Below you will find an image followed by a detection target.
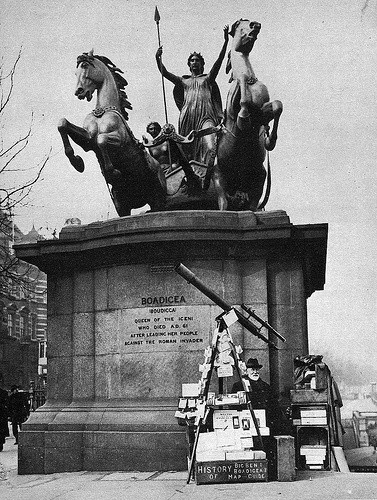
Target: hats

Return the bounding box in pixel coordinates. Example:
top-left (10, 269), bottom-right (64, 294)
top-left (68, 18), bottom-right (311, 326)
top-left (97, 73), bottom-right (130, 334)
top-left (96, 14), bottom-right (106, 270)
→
top-left (246, 358), bottom-right (263, 369)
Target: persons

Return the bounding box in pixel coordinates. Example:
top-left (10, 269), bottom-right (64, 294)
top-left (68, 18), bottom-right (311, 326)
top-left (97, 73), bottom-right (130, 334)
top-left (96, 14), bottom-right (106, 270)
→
top-left (230, 358), bottom-right (278, 432)
top-left (0, 388), bottom-right (9, 452)
top-left (142, 122), bottom-right (190, 171)
top-left (155, 23), bottom-right (230, 209)
top-left (8, 385), bottom-right (31, 445)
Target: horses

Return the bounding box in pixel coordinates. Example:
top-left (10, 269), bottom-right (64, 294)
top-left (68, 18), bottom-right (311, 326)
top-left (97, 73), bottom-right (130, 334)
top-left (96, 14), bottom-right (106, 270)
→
top-left (57, 47), bottom-right (168, 218)
top-left (215, 17), bottom-right (283, 212)
